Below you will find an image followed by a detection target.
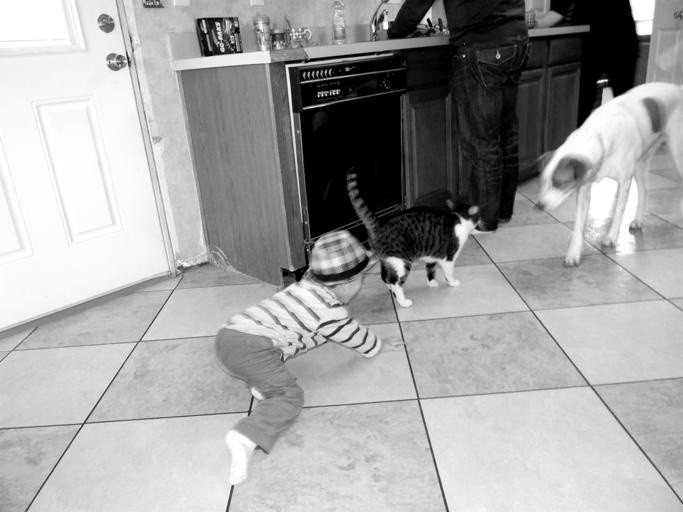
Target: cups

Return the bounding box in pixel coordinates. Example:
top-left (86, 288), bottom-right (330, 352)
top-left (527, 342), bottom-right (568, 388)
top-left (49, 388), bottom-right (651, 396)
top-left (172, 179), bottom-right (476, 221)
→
top-left (524, 11), bottom-right (535, 28)
top-left (285, 27), bottom-right (312, 49)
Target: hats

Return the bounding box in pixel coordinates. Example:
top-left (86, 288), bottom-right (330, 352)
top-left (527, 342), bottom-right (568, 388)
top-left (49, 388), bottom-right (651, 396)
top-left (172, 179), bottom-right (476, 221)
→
top-left (301, 230), bottom-right (380, 287)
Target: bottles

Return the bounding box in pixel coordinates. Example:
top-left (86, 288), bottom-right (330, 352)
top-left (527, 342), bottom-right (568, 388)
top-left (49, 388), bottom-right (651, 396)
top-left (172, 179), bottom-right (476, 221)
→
top-left (331, 1), bottom-right (347, 43)
top-left (251, 12), bottom-right (272, 52)
top-left (269, 27), bottom-right (285, 50)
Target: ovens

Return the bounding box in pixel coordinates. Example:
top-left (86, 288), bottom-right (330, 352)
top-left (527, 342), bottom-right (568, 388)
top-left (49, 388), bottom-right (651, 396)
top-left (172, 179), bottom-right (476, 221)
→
top-left (285, 54), bottom-right (410, 269)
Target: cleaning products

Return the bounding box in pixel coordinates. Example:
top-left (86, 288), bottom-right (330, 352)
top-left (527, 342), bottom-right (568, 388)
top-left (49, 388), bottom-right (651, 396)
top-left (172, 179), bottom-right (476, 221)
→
top-left (333, 0), bottom-right (347, 45)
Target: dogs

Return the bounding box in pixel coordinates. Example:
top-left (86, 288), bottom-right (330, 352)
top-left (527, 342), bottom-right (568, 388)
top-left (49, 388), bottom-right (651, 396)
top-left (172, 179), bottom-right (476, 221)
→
top-left (533, 82), bottom-right (683, 267)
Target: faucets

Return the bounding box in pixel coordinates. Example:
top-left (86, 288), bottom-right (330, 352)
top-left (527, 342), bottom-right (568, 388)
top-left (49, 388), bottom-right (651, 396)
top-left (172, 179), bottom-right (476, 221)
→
top-left (369, 0), bottom-right (390, 41)
top-left (377, 10), bottom-right (388, 31)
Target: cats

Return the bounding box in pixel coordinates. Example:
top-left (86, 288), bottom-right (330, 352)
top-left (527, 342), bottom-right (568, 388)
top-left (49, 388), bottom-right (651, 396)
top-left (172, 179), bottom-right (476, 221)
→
top-left (346, 165), bottom-right (487, 307)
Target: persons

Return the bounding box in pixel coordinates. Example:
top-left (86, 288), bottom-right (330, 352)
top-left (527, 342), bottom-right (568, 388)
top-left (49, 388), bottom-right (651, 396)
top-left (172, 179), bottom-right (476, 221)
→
top-left (215, 231), bottom-right (406, 485)
top-left (386, 0), bottom-right (531, 236)
top-left (535, 2), bottom-right (640, 126)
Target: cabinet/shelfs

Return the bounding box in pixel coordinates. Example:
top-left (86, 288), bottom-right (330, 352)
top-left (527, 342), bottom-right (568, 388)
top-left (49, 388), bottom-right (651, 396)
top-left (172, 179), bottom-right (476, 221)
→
top-left (403, 87), bottom-right (454, 207)
top-left (452, 35), bottom-right (587, 194)
